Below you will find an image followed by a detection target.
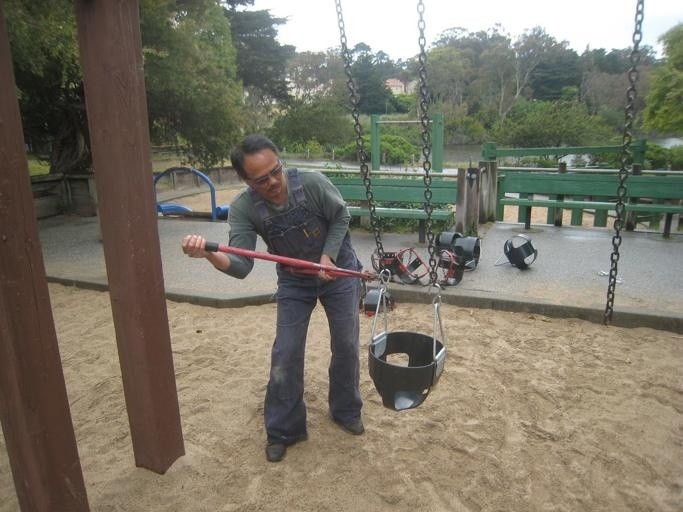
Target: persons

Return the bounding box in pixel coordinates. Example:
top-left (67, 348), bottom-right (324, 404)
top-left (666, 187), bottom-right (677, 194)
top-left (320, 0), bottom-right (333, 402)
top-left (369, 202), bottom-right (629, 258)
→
top-left (180, 133), bottom-right (365, 463)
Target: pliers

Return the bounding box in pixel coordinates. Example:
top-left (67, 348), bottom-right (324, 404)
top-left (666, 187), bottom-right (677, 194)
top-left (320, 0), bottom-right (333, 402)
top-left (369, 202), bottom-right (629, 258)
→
top-left (204, 242), bottom-right (405, 285)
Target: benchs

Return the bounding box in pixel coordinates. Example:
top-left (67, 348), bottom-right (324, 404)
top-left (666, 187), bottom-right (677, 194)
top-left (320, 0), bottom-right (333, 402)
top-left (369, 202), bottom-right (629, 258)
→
top-left (329, 173), bottom-right (460, 243)
top-left (496, 170), bottom-right (681, 238)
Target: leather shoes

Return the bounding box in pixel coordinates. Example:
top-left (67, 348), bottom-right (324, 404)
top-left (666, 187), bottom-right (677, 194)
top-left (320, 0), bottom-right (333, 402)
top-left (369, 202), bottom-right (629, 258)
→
top-left (265, 436), bottom-right (288, 462)
top-left (331, 414), bottom-right (367, 435)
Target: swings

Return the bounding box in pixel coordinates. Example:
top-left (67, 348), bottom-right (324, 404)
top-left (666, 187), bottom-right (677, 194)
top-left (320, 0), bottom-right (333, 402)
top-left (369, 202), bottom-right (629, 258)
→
top-left (333, 0), bottom-right (447, 410)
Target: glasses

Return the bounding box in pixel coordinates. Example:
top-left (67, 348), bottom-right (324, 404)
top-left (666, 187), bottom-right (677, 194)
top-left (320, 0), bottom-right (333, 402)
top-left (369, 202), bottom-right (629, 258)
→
top-left (244, 165), bottom-right (284, 186)
